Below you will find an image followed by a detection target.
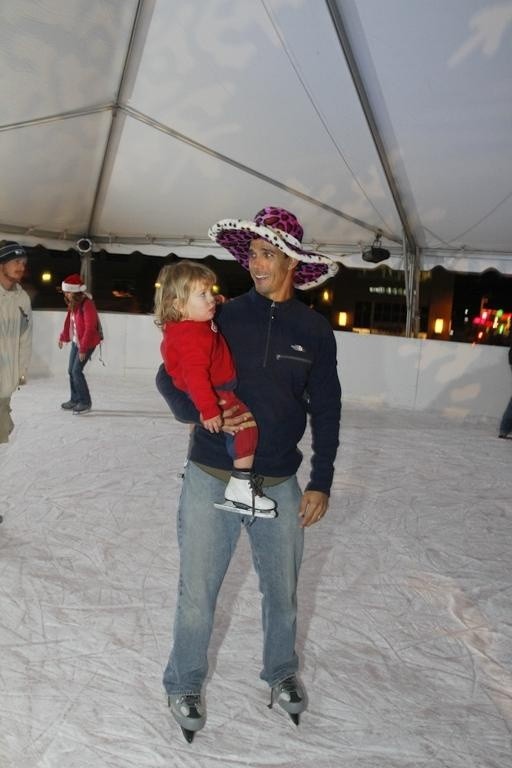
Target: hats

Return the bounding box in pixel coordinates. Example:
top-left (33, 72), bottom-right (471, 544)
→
top-left (0, 238), bottom-right (28, 268)
top-left (206, 204), bottom-right (344, 295)
top-left (61, 272), bottom-right (86, 294)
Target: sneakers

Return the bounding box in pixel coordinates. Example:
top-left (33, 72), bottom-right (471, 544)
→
top-left (168, 694), bottom-right (207, 731)
top-left (271, 677), bottom-right (309, 715)
top-left (62, 395), bottom-right (93, 412)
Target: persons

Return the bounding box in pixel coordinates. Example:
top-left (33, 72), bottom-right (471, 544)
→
top-left (1, 237), bottom-right (34, 527)
top-left (156, 258), bottom-right (281, 513)
top-left (155, 202), bottom-right (344, 732)
top-left (56, 271), bottom-right (104, 414)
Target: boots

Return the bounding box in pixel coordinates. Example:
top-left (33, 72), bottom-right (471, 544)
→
top-left (224, 468), bottom-right (277, 510)
